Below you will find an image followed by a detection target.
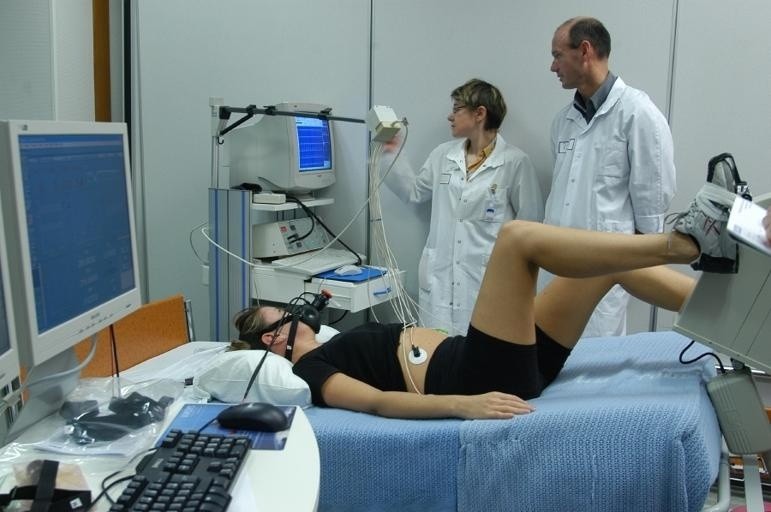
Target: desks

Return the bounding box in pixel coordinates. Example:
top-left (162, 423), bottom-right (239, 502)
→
top-left (0, 403), bottom-right (322, 512)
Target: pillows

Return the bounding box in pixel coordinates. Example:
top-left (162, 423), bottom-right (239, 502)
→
top-left (192, 323), bottom-right (341, 410)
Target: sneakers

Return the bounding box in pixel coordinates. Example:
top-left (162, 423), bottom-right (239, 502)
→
top-left (664, 154), bottom-right (739, 274)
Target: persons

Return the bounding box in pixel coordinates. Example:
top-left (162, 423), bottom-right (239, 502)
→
top-left (231, 152), bottom-right (753, 419)
top-left (534, 15), bottom-right (677, 340)
top-left (761, 203), bottom-right (770, 241)
top-left (376, 79), bottom-right (545, 333)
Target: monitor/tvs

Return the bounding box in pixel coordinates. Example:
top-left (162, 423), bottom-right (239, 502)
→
top-left (222, 101), bottom-right (336, 204)
top-left (1, 117), bottom-right (144, 444)
top-left (1, 216), bottom-right (19, 449)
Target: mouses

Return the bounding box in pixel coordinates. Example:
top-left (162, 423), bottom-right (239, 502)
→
top-left (217, 403), bottom-right (290, 431)
top-left (334, 264), bottom-right (361, 275)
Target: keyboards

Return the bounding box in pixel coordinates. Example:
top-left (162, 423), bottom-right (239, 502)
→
top-left (108, 428), bottom-right (251, 511)
top-left (271, 247), bottom-right (366, 276)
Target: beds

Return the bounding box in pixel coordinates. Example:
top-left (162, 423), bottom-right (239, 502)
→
top-left (28, 295), bottom-right (732, 511)
